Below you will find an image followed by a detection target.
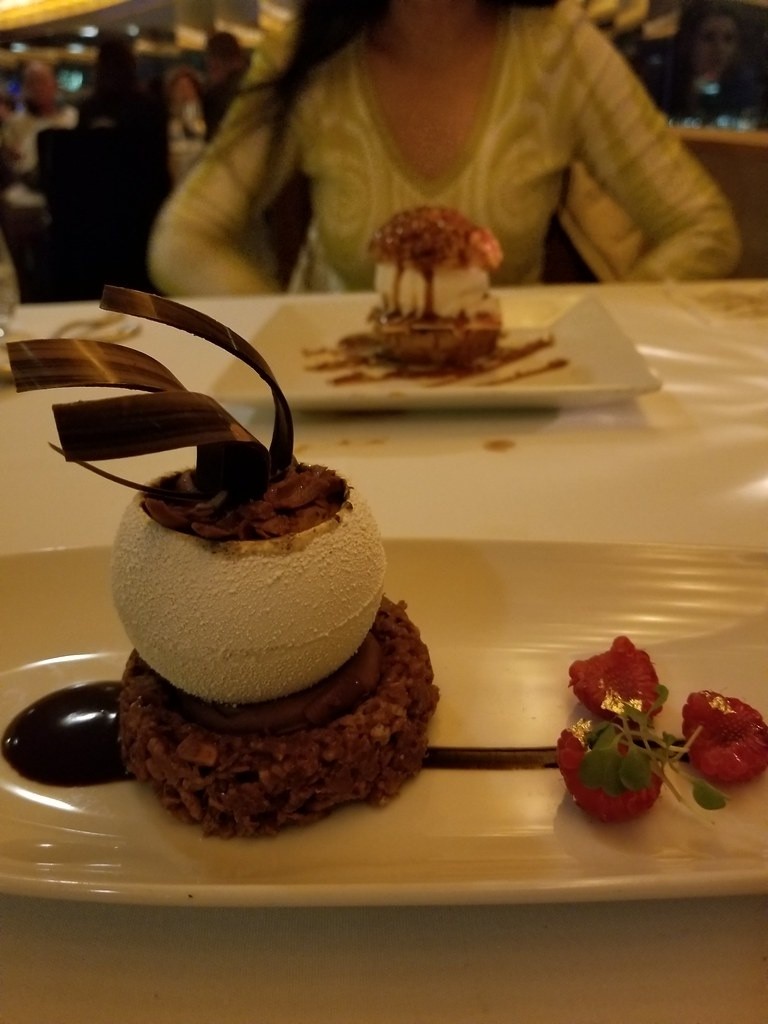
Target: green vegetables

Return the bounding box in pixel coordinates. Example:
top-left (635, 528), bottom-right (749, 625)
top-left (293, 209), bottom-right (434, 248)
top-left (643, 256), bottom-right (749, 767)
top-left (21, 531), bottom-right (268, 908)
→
top-left (576, 680), bottom-right (732, 813)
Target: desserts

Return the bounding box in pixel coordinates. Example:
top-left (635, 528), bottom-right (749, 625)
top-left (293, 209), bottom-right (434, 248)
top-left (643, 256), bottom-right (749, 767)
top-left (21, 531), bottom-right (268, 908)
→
top-left (6, 285), bottom-right (441, 841)
top-left (366, 204), bottom-right (502, 368)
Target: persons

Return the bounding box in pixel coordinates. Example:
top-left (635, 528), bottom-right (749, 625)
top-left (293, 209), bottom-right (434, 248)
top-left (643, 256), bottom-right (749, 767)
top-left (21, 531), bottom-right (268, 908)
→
top-left (1, 31), bottom-right (251, 303)
top-left (662, 1), bottom-right (768, 129)
top-left (148, 1), bottom-right (743, 299)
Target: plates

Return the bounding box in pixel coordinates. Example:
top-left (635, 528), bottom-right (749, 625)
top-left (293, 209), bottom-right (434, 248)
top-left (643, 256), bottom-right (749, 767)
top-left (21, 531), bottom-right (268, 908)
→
top-left (0, 534), bottom-right (767, 906)
top-left (209, 289), bottom-right (663, 416)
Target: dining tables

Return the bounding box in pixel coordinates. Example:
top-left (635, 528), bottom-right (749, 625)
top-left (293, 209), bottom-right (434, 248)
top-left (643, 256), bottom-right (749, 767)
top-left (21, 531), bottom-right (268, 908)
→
top-left (0, 277), bottom-right (768, 1024)
